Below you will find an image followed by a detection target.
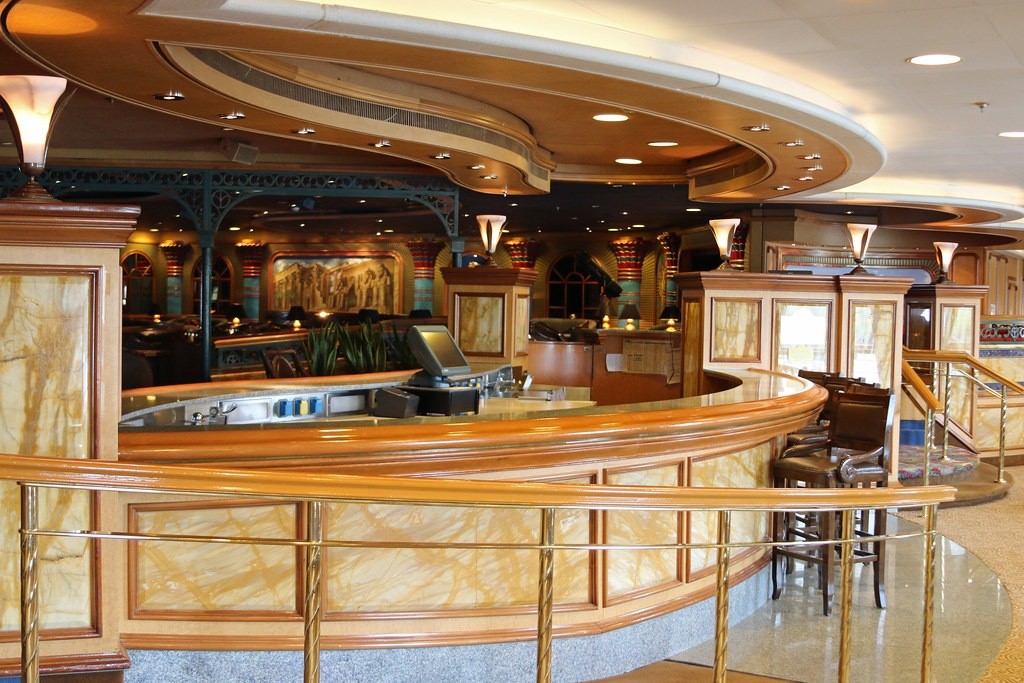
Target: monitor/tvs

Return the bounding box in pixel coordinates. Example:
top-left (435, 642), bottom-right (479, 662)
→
top-left (406, 324), bottom-right (472, 377)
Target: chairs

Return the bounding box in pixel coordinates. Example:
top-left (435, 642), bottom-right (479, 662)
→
top-left (260, 348), bottom-right (312, 381)
top-left (770, 369), bottom-right (897, 615)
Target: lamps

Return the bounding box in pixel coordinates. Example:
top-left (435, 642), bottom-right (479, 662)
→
top-left (707, 218), bottom-right (744, 273)
top-left (842, 222), bottom-right (877, 277)
top-left (475, 214), bottom-right (508, 268)
top-left (0, 75), bottom-right (78, 205)
top-left (931, 241), bottom-right (959, 283)
